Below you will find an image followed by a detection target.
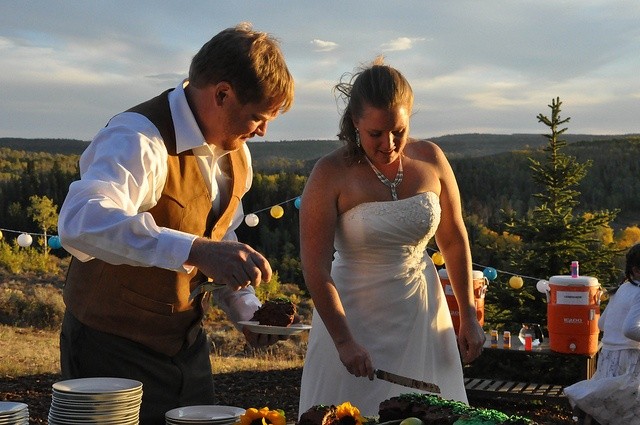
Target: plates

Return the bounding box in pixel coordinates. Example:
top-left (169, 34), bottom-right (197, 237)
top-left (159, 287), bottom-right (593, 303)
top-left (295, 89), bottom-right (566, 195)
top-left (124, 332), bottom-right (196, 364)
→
top-left (48, 376), bottom-right (144, 425)
top-left (164, 405), bottom-right (246, 425)
top-left (237, 320), bottom-right (313, 336)
top-left (0, 401), bottom-right (30, 425)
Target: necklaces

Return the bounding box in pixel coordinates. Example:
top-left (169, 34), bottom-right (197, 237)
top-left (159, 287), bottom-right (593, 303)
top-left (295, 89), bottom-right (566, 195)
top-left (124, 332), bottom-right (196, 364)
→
top-left (358, 143), bottom-right (411, 203)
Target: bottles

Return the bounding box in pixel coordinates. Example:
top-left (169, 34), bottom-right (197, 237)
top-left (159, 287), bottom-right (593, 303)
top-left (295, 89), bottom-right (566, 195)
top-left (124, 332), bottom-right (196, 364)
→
top-left (571, 260), bottom-right (579, 277)
top-left (525, 333), bottom-right (533, 351)
top-left (491, 330), bottom-right (498, 348)
top-left (503, 331), bottom-right (511, 350)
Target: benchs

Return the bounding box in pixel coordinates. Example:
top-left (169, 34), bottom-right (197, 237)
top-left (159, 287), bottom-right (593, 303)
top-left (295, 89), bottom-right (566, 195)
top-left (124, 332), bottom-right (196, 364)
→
top-left (462, 375), bottom-right (568, 400)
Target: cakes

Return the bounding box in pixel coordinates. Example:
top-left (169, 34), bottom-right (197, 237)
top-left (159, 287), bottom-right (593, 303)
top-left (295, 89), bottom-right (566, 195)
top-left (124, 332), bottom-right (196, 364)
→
top-left (298, 391), bottom-right (538, 425)
top-left (249, 296), bottom-right (298, 330)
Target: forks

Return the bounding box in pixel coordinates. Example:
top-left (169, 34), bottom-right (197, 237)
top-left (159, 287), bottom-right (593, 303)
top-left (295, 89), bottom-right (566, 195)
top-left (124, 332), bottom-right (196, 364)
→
top-left (188, 281), bottom-right (229, 303)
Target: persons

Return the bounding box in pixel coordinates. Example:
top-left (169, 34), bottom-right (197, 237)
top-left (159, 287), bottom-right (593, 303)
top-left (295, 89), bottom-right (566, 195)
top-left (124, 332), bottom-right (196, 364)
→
top-left (598, 242), bottom-right (640, 425)
top-left (298, 56), bottom-right (488, 425)
top-left (57, 22), bottom-right (296, 425)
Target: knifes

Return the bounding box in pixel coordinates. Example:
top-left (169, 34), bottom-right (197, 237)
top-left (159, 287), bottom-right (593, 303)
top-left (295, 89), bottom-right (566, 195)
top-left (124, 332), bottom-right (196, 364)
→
top-left (374, 368), bottom-right (440, 394)
